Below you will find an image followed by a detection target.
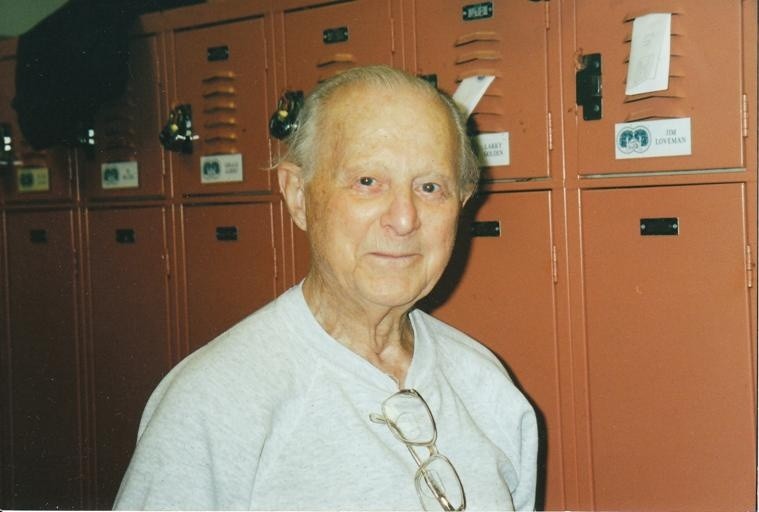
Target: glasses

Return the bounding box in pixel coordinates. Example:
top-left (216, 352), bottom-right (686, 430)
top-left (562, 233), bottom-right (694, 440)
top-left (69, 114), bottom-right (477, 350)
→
top-left (370, 389), bottom-right (466, 511)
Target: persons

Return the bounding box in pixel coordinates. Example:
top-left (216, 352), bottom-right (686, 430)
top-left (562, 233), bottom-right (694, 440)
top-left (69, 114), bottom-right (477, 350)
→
top-left (111, 63), bottom-right (539, 511)
